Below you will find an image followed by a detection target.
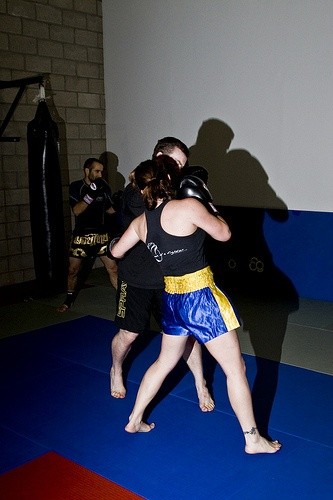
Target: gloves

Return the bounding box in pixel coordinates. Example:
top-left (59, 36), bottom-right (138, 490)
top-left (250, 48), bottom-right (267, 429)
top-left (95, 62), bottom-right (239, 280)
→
top-left (190, 164), bottom-right (210, 187)
top-left (103, 236), bottom-right (123, 260)
top-left (179, 176), bottom-right (213, 207)
top-left (84, 177), bottom-right (111, 206)
top-left (111, 189), bottom-right (123, 204)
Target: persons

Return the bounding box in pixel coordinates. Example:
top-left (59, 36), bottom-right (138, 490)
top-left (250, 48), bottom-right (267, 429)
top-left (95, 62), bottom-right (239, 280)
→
top-left (104, 136), bottom-right (282, 453)
top-left (56, 158), bottom-right (118, 311)
